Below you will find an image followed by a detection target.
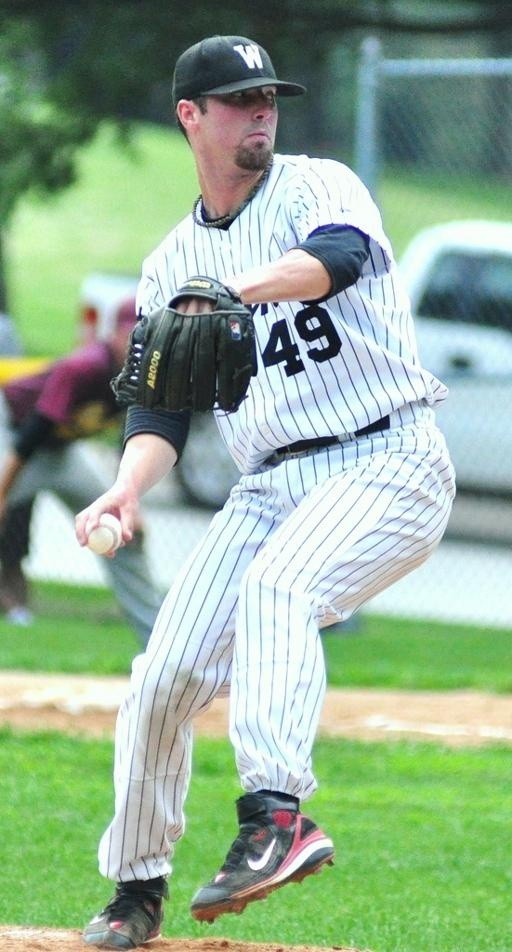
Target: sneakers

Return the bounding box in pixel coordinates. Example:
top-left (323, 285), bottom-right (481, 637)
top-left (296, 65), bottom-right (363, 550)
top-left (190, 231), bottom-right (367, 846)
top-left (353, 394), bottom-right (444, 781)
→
top-left (84, 875), bottom-right (169, 952)
top-left (188, 791), bottom-right (333, 924)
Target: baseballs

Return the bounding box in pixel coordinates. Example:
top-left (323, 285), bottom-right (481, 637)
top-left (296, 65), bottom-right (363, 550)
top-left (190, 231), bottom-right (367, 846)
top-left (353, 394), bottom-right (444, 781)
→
top-left (87, 515), bottom-right (124, 557)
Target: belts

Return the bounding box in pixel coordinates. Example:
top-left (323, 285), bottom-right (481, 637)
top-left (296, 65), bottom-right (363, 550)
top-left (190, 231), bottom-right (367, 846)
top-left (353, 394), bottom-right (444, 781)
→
top-left (273, 416), bottom-right (390, 455)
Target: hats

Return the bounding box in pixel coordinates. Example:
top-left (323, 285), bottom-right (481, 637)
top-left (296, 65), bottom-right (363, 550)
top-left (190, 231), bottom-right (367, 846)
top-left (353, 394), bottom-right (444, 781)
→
top-left (175, 35), bottom-right (310, 97)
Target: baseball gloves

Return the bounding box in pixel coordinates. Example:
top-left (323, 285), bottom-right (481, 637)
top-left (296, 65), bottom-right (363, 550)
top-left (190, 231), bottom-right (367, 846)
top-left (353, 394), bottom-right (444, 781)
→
top-left (110, 278), bottom-right (258, 414)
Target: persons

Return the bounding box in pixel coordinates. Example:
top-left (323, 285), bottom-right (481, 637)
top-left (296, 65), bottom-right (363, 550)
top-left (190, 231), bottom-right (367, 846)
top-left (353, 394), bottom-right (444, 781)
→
top-left (70, 304), bottom-right (103, 352)
top-left (73, 34), bottom-right (457, 952)
top-left (0, 296), bottom-right (165, 647)
top-left (1, 301), bottom-right (28, 358)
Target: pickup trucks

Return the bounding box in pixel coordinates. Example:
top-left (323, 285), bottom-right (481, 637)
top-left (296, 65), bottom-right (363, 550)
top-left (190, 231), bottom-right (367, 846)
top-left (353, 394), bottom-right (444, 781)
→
top-left (72, 214), bottom-right (511, 513)
